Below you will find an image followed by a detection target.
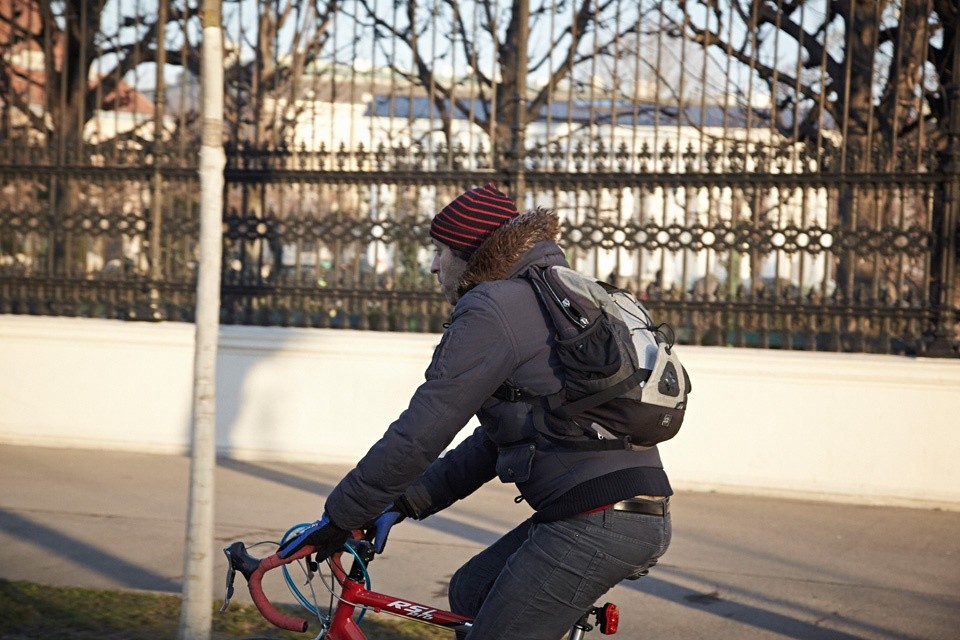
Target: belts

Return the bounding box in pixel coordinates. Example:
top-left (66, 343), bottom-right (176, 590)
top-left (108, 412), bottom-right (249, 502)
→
top-left (613, 494), bottom-right (670, 515)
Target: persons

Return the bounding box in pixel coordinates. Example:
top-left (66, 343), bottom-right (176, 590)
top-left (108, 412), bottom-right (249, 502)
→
top-left (272, 185), bottom-right (675, 639)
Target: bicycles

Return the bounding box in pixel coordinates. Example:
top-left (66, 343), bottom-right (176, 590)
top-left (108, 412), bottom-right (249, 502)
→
top-left (217, 527), bottom-right (659, 640)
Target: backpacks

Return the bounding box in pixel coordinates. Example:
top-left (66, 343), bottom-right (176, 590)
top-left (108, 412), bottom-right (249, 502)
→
top-left (525, 263), bottom-right (692, 443)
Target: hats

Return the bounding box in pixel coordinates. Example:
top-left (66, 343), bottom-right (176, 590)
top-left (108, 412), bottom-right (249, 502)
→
top-left (430, 182), bottom-right (518, 258)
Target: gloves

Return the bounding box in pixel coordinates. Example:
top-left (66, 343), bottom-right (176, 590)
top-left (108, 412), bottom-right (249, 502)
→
top-left (370, 496), bottom-right (414, 554)
top-left (277, 513), bottom-right (350, 564)
